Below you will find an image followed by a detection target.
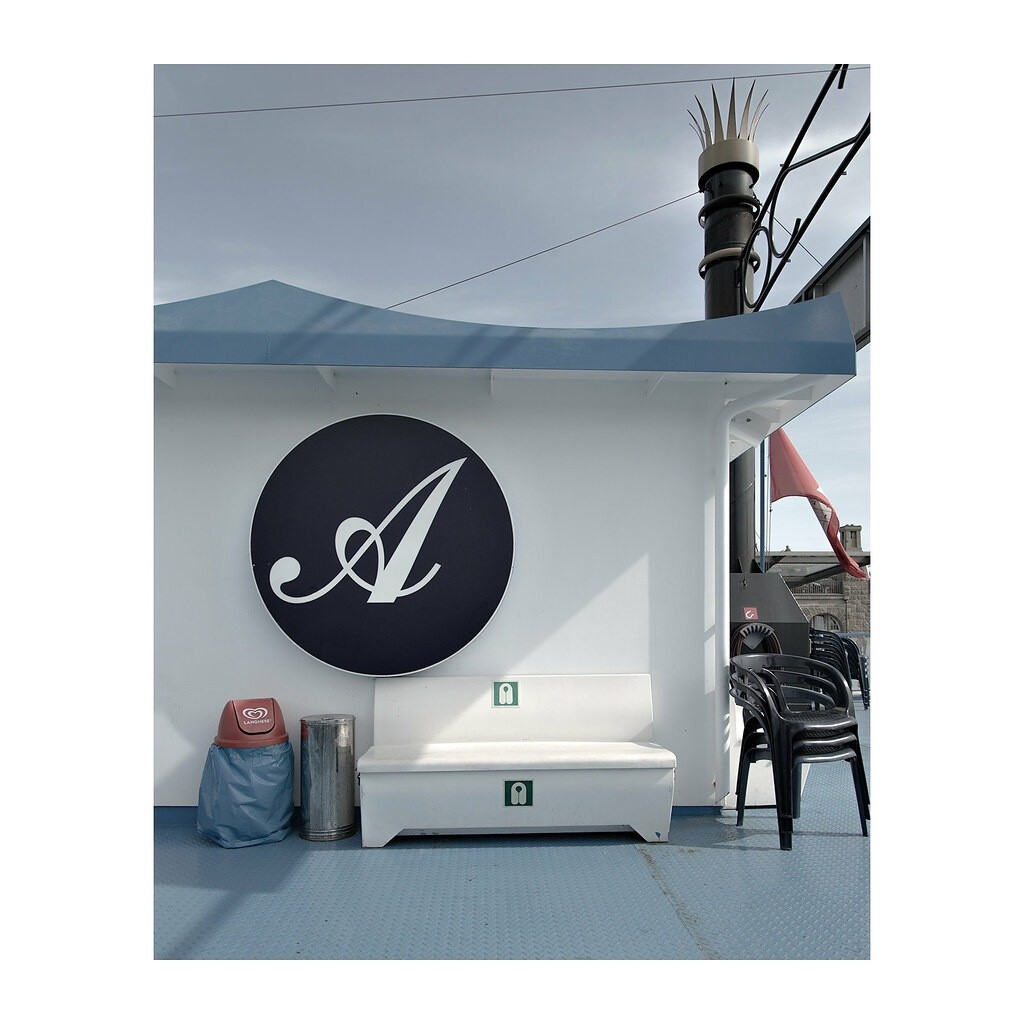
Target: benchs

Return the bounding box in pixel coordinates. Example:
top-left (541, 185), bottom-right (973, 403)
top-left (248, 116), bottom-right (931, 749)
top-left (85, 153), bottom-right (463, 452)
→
top-left (359, 671), bottom-right (675, 843)
top-left (730, 695), bottom-right (811, 807)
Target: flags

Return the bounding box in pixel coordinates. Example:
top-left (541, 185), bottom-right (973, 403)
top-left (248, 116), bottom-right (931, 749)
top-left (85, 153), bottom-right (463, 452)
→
top-left (769, 428), bottom-right (866, 578)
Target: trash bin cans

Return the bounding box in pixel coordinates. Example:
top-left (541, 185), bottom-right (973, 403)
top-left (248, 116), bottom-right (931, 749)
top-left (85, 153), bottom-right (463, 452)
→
top-left (209, 698), bottom-right (298, 844)
top-left (297, 714), bottom-right (358, 843)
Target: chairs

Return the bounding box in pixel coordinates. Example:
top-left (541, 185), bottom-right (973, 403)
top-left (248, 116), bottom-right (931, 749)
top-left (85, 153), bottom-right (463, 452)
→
top-left (727, 625), bottom-right (870, 850)
